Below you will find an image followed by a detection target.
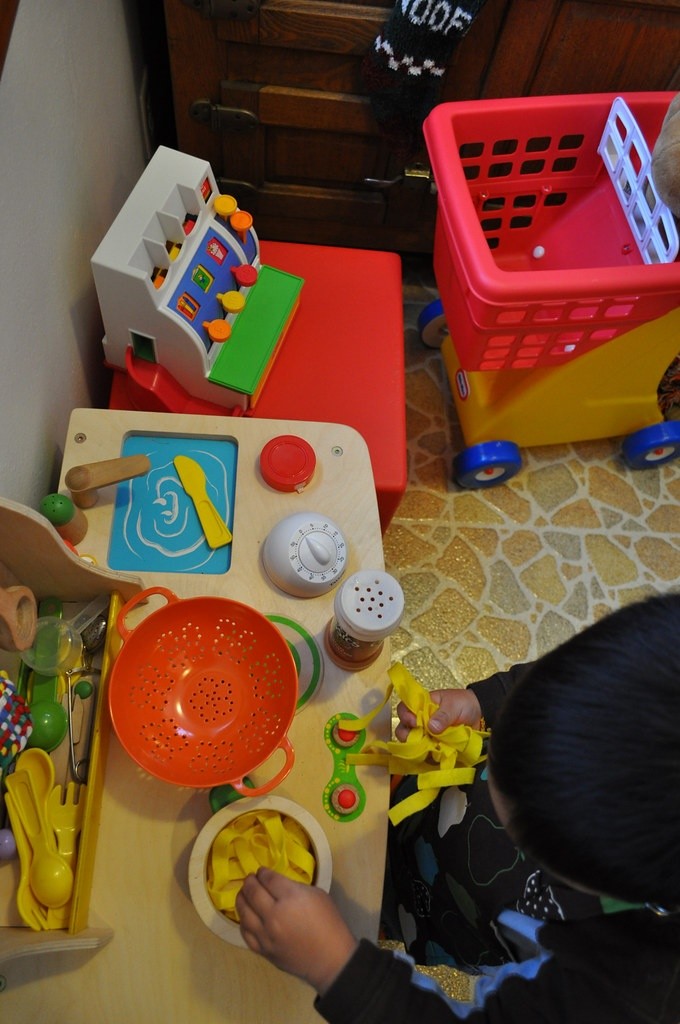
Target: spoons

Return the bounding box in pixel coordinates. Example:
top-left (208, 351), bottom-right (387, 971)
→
top-left (27, 599), bottom-right (68, 753)
top-left (5, 749), bottom-right (73, 908)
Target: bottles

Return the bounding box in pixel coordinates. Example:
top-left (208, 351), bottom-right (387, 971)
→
top-left (323, 569), bottom-right (405, 672)
top-left (40, 494), bottom-right (89, 548)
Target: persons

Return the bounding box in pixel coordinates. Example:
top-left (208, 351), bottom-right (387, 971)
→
top-left (235, 592), bottom-right (680, 1024)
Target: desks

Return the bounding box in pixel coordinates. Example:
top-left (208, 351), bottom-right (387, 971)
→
top-left (0, 409), bottom-right (388, 1024)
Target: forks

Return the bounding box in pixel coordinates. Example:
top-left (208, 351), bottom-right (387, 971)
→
top-left (48, 781), bottom-right (87, 930)
top-left (4, 793), bottom-right (48, 932)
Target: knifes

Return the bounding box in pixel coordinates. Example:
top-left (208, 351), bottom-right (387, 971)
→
top-left (173, 455), bottom-right (232, 550)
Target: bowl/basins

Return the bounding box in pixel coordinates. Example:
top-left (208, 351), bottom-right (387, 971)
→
top-left (188, 794), bottom-right (333, 949)
